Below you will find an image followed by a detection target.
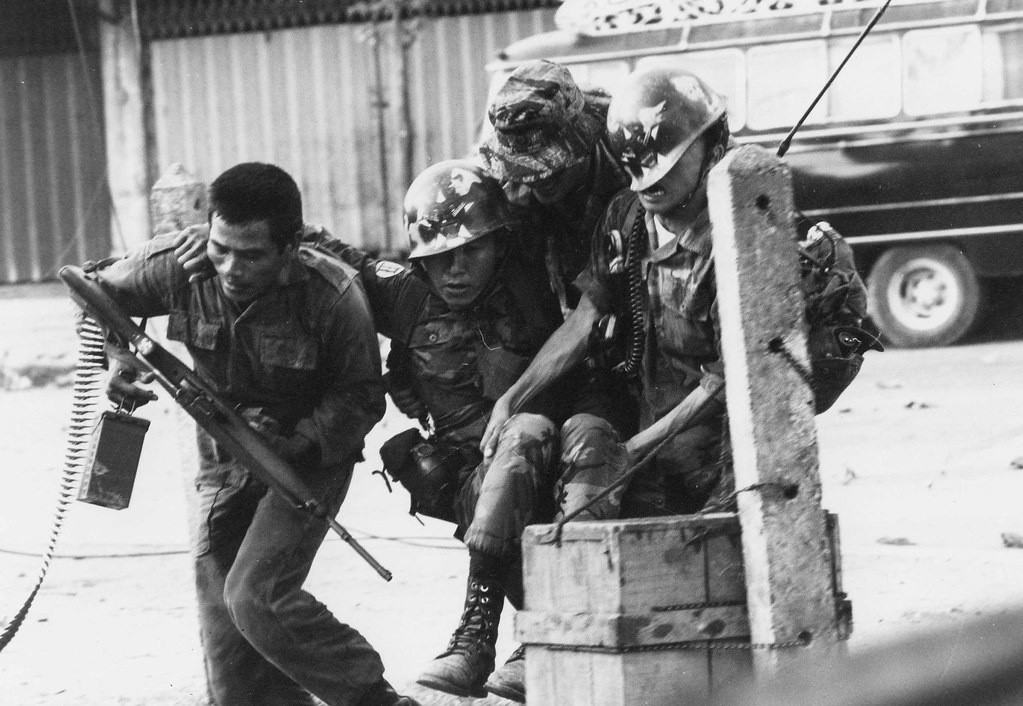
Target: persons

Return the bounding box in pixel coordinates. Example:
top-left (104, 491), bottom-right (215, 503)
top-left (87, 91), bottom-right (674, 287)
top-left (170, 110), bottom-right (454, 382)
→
top-left (467, 59), bottom-right (632, 314)
top-left (96, 161), bottom-right (420, 706)
top-left (173, 161), bottom-right (631, 703)
top-left (477, 62), bottom-right (868, 519)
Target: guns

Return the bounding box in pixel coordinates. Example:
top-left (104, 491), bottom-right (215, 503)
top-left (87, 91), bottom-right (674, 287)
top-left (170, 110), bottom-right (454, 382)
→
top-left (58, 264), bottom-right (394, 583)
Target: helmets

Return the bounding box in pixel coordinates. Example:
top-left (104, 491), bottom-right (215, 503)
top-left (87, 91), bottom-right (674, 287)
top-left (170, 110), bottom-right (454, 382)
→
top-left (401, 158), bottom-right (520, 261)
top-left (606, 66), bottom-right (726, 190)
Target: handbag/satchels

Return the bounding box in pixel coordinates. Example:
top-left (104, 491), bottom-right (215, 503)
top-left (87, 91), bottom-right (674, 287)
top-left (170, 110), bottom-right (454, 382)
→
top-left (796, 220), bottom-right (883, 416)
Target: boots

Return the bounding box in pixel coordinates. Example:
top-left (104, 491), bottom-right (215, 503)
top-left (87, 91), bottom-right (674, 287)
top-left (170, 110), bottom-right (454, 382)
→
top-left (485, 642), bottom-right (527, 703)
top-left (417, 575), bottom-right (506, 699)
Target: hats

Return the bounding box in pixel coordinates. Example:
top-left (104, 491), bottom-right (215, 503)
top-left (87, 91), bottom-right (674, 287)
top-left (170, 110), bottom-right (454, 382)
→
top-left (478, 61), bottom-right (612, 181)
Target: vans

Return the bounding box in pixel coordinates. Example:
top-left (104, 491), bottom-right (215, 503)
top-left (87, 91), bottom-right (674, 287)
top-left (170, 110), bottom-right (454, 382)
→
top-left (478, 0), bottom-right (1023, 349)
